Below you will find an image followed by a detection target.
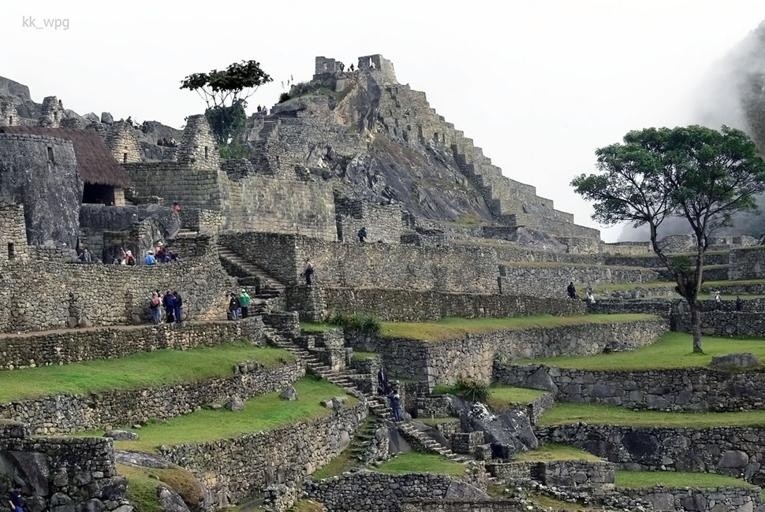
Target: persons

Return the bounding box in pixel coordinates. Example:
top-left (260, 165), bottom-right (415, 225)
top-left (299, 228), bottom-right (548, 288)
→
top-left (378, 368), bottom-right (389, 396)
top-left (390, 390), bottom-right (403, 424)
top-left (567, 281), bottom-right (576, 299)
top-left (714, 290), bottom-right (722, 311)
top-left (586, 288), bottom-right (595, 304)
top-left (0, 61), bottom-right (368, 327)
